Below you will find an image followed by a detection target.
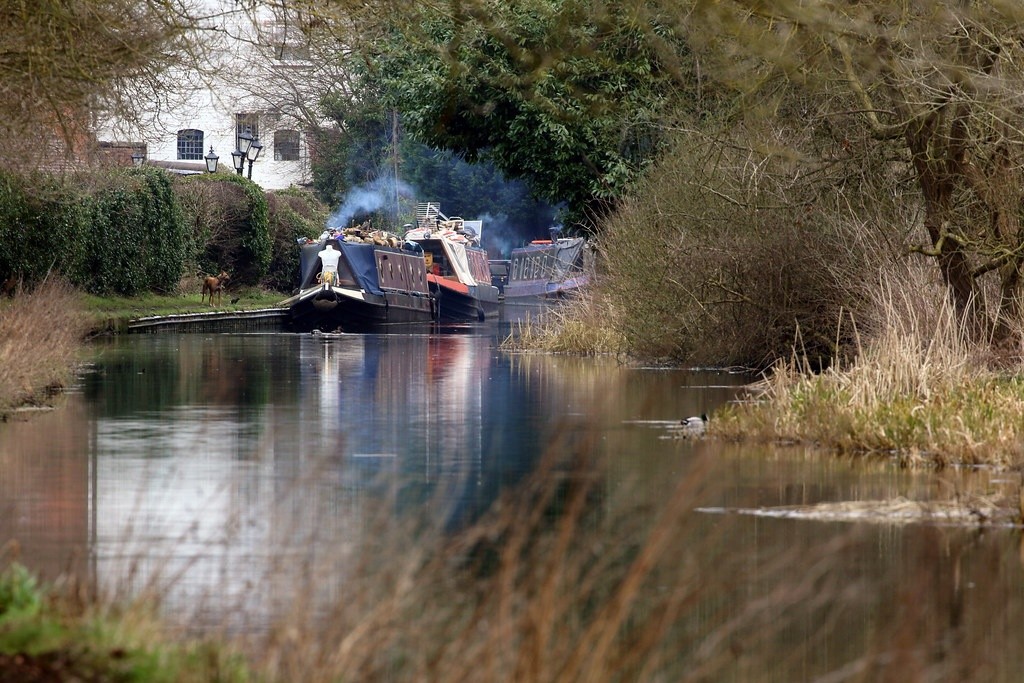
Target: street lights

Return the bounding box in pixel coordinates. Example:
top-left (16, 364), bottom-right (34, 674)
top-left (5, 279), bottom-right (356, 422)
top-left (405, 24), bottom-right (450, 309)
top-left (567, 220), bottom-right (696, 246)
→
top-left (238, 125), bottom-right (254, 176)
top-left (232, 148), bottom-right (242, 175)
top-left (247, 133), bottom-right (263, 180)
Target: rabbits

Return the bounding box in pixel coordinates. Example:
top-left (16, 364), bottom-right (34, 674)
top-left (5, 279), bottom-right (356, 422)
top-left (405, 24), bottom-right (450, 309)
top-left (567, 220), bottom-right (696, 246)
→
top-left (230, 297), bottom-right (240, 304)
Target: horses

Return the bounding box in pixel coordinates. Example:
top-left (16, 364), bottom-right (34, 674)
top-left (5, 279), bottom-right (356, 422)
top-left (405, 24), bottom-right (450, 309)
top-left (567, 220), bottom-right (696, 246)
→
top-left (201, 269), bottom-right (230, 308)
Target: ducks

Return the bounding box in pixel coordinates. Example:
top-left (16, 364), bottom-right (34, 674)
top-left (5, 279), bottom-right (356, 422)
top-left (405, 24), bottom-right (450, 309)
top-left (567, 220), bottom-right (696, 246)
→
top-left (332, 326), bottom-right (343, 334)
top-left (681, 413), bottom-right (710, 427)
top-left (310, 325), bottom-right (323, 335)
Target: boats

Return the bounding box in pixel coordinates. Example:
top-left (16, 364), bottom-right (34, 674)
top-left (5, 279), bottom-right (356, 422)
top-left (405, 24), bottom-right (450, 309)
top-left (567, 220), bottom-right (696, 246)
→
top-left (497, 236), bottom-right (595, 328)
top-left (286, 201), bottom-right (502, 332)
top-left (487, 258), bottom-right (511, 297)
